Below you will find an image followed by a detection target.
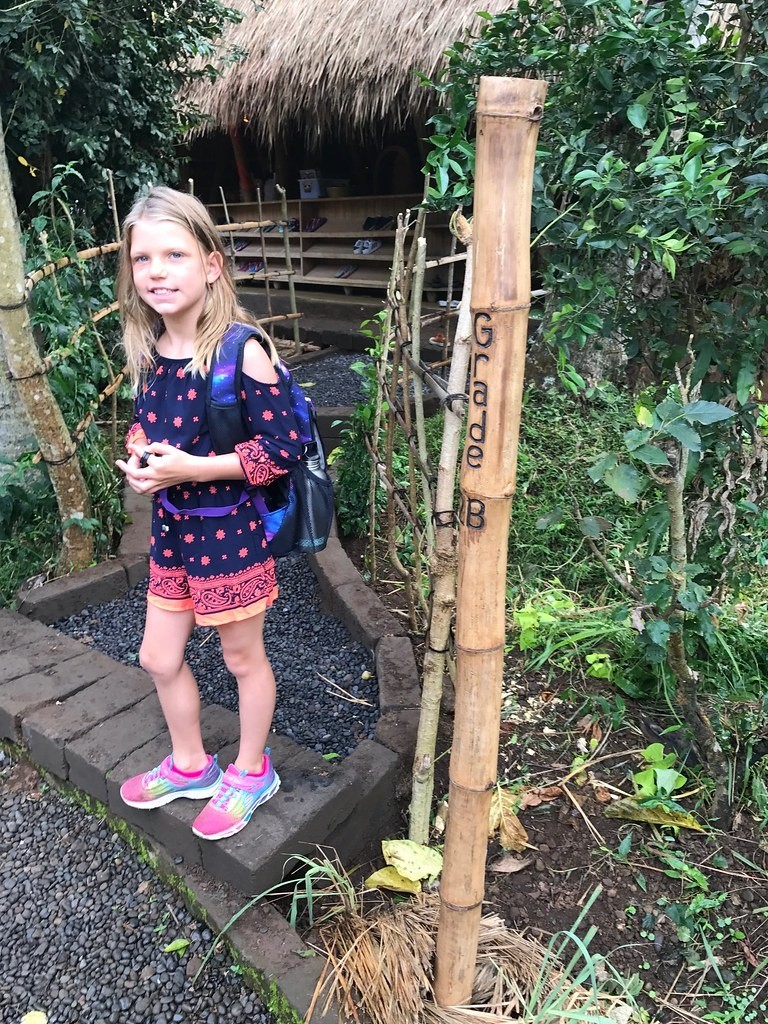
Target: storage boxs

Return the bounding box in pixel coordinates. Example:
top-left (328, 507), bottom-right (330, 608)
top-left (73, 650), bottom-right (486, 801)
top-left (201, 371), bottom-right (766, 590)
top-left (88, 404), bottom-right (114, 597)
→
top-left (299, 168), bottom-right (321, 179)
top-left (297, 178), bottom-right (345, 198)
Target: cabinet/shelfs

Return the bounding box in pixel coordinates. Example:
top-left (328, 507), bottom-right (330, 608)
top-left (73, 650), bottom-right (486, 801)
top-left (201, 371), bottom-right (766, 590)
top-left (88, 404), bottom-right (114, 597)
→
top-left (202, 194), bottom-right (465, 313)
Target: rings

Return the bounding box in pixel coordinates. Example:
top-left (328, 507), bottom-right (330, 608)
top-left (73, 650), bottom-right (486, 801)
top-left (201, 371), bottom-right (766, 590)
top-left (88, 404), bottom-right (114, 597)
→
top-left (139, 451), bottom-right (151, 463)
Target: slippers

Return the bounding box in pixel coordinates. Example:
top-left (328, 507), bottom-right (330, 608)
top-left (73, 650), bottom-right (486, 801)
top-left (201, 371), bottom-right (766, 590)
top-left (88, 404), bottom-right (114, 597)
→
top-left (234, 239), bottom-right (251, 251)
top-left (304, 216), bottom-right (327, 231)
top-left (334, 262), bottom-right (359, 278)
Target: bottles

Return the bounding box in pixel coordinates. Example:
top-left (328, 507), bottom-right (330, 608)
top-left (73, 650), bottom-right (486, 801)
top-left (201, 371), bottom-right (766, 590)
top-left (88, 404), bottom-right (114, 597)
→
top-left (301, 440), bottom-right (331, 482)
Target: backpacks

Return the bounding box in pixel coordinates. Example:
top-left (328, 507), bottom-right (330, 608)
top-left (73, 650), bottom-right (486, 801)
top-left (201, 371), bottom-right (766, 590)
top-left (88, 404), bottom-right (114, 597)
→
top-left (158, 320), bottom-right (335, 557)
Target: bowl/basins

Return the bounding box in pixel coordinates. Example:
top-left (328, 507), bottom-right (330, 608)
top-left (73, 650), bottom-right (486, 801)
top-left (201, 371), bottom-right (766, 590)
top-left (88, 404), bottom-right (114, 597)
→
top-left (326, 187), bottom-right (346, 197)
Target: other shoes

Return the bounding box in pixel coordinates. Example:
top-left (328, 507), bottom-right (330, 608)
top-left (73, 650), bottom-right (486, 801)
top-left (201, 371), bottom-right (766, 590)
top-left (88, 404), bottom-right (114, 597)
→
top-left (362, 214), bottom-right (416, 231)
top-left (258, 219), bottom-right (276, 232)
top-left (278, 217), bottom-right (298, 232)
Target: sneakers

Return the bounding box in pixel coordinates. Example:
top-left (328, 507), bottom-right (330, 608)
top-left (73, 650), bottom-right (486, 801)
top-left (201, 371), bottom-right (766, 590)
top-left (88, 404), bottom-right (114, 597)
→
top-left (237, 260), bottom-right (264, 272)
top-left (191, 746), bottom-right (281, 840)
top-left (119, 750), bottom-right (224, 809)
top-left (353, 238), bottom-right (381, 253)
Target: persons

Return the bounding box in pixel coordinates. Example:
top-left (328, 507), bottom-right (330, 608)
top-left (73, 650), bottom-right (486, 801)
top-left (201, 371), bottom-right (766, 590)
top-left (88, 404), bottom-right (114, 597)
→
top-left (115, 188), bottom-right (303, 841)
